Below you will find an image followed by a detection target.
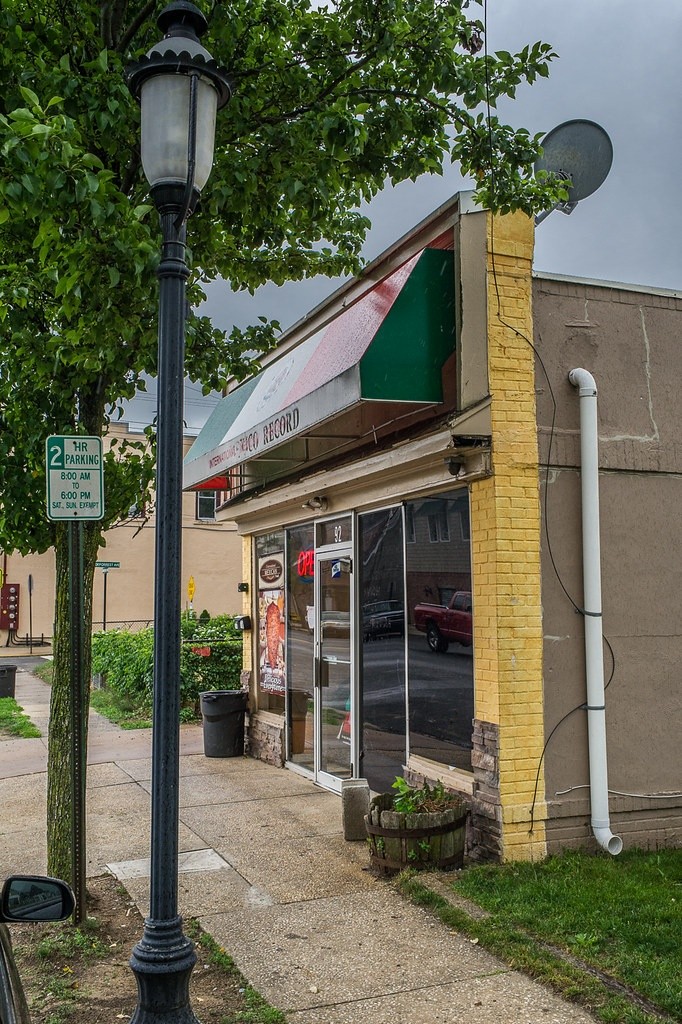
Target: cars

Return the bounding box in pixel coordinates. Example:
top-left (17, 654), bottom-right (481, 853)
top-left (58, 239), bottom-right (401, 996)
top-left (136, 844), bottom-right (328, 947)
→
top-left (361, 600), bottom-right (405, 641)
top-left (320, 611), bottom-right (350, 638)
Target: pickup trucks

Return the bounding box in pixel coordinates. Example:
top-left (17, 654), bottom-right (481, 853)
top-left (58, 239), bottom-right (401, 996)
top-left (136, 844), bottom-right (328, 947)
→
top-left (414, 591), bottom-right (473, 653)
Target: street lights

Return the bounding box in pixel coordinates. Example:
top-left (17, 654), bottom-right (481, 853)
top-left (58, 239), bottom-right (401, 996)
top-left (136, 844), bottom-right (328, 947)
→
top-left (129, 2), bottom-right (231, 1024)
top-left (102, 569), bottom-right (109, 631)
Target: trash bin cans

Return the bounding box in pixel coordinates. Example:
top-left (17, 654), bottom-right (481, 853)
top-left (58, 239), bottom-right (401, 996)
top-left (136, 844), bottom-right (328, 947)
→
top-left (288, 688), bottom-right (309, 754)
top-left (199, 689), bottom-right (249, 758)
top-left (0, 664), bottom-right (18, 700)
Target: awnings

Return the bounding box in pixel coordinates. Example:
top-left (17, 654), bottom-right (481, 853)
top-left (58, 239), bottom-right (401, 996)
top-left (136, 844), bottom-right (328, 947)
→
top-left (181, 249), bottom-right (455, 492)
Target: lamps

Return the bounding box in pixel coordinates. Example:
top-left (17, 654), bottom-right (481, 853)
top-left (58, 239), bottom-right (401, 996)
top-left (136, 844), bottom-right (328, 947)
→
top-left (303, 496), bottom-right (329, 512)
top-left (235, 616), bottom-right (250, 630)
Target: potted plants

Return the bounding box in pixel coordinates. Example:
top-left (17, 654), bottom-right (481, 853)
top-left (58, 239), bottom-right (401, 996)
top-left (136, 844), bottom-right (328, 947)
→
top-left (364, 773), bottom-right (469, 879)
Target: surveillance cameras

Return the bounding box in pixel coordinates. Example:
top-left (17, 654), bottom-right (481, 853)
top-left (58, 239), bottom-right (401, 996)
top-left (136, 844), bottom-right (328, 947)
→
top-left (444, 457), bottom-right (466, 476)
